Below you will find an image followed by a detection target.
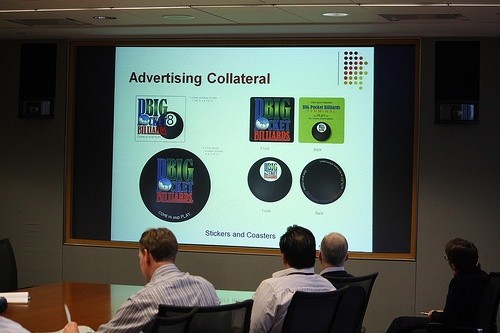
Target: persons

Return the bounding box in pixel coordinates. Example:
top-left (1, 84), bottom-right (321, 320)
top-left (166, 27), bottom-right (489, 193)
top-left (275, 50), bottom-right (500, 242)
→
top-left (233, 225), bottom-right (338, 333)
top-left (386, 238), bottom-right (489, 333)
top-left (0, 316), bottom-right (31, 333)
top-left (64, 228), bottom-right (220, 333)
top-left (317, 232), bottom-right (356, 288)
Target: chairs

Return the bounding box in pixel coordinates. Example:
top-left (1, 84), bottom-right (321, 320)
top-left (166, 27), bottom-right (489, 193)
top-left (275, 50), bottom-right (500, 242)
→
top-left (281, 288), bottom-right (345, 333)
top-left (150, 299), bottom-right (254, 333)
top-left (426, 272), bottom-right (500, 333)
top-left (0, 238), bottom-right (18, 293)
top-left (326, 272), bottom-right (379, 333)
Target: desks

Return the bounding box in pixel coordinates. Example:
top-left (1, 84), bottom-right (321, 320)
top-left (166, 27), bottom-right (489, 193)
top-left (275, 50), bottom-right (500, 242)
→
top-left (0, 281), bottom-right (144, 333)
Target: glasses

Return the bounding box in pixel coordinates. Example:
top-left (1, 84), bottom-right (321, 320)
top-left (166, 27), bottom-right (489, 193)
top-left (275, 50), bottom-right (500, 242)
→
top-left (444, 255), bottom-right (449, 261)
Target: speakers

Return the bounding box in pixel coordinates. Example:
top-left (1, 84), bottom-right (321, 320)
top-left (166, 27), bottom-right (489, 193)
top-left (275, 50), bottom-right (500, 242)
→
top-left (18, 42), bottom-right (57, 120)
top-left (434, 41), bottom-right (480, 125)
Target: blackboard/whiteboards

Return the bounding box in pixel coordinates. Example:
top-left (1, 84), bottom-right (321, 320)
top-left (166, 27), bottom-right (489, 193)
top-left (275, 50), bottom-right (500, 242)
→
top-left (63, 37), bottom-right (419, 260)
